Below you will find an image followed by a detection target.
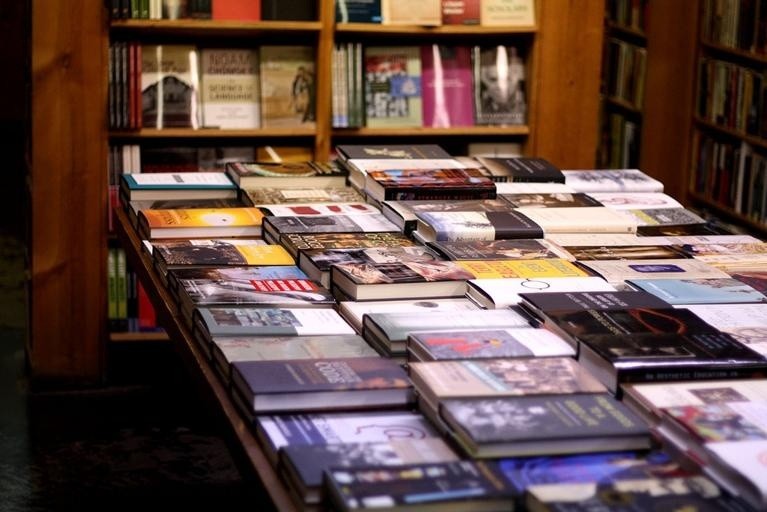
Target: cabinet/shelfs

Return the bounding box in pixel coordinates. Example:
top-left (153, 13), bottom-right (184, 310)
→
top-left (25, 0), bottom-right (767, 376)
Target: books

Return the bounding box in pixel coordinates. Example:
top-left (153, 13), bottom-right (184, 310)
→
top-left (593, 2), bottom-right (647, 168)
top-left (105, 0), bottom-right (537, 331)
top-left (690, 1), bottom-right (767, 238)
top-left (120, 144), bottom-right (767, 512)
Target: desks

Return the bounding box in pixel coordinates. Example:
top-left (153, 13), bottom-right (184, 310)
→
top-left (112, 205), bottom-right (767, 512)
